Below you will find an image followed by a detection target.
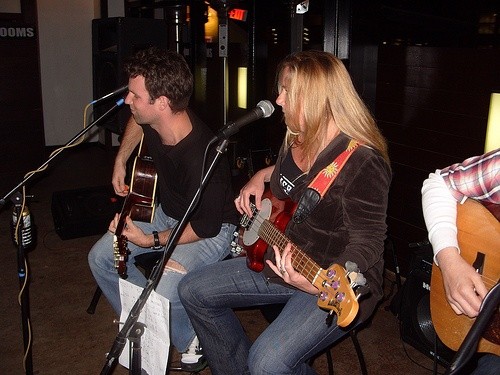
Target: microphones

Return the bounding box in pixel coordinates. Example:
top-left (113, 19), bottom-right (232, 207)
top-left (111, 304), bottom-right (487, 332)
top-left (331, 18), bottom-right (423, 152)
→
top-left (210, 100), bottom-right (275, 144)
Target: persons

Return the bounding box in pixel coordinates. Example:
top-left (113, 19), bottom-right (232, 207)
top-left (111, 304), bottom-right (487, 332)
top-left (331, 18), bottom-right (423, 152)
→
top-left (86, 47), bottom-right (239, 375)
top-left (177, 50), bottom-right (393, 375)
top-left (420, 147), bottom-right (500, 375)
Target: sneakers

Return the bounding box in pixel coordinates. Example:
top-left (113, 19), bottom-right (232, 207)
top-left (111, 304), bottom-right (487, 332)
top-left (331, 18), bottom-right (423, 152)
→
top-left (180, 336), bottom-right (208, 371)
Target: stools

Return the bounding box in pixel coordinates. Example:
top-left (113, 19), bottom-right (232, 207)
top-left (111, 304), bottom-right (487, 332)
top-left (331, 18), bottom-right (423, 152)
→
top-left (258, 303), bottom-right (378, 375)
top-left (84, 251), bottom-right (238, 314)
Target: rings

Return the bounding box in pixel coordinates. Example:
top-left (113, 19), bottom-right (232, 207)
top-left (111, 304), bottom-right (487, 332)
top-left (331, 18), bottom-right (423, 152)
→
top-left (281, 270), bottom-right (286, 274)
top-left (236, 195), bottom-right (240, 199)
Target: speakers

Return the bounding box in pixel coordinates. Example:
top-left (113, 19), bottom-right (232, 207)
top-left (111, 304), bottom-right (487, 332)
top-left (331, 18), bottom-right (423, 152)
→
top-left (91, 16), bottom-right (170, 134)
top-left (398, 256), bottom-right (457, 368)
top-left (52, 184), bottom-right (127, 241)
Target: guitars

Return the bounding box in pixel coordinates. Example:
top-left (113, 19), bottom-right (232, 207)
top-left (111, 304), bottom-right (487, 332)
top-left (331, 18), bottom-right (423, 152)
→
top-left (430, 198), bottom-right (500, 356)
top-left (230, 186), bottom-right (360, 329)
top-left (113, 155), bottom-right (158, 280)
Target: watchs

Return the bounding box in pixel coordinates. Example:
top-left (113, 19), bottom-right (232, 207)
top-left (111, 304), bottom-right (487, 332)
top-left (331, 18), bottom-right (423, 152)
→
top-left (151, 231), bottom-right (162, 250)
